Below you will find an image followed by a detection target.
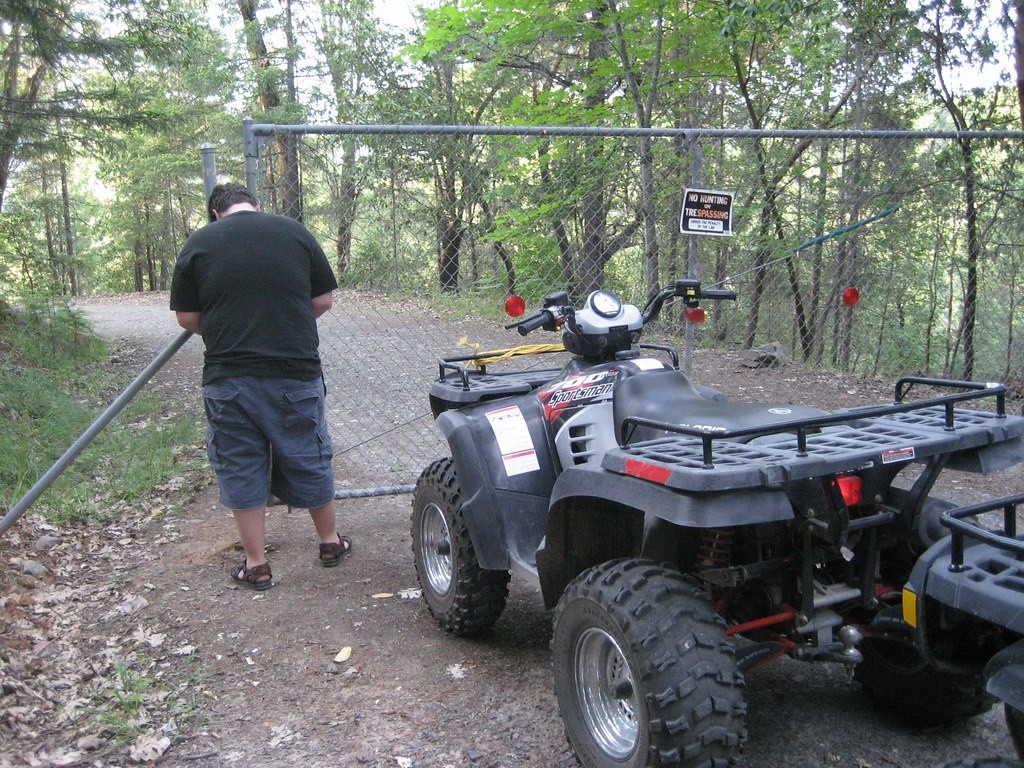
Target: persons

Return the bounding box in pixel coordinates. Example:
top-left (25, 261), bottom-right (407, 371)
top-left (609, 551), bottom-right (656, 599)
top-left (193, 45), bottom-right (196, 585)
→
top-left (169, 182), bottom-right (353, 591)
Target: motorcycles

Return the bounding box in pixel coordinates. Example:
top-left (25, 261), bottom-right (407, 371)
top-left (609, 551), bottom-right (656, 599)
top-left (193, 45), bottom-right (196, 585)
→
top-left (408, 276), bottom-right (1024, 768)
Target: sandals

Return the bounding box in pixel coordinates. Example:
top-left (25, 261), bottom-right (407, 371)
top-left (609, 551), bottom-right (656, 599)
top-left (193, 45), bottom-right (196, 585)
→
top-left (231, 558), bottom-right (273, 590)
top-left (319, 532), bottom-right (352, 568)
